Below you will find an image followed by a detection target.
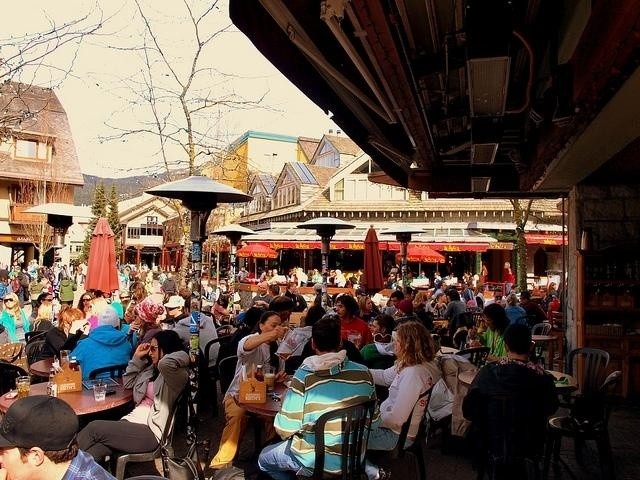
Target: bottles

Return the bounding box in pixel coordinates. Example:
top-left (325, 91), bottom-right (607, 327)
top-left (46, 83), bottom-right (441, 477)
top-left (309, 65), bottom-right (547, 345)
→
top-left (48, 368), bottom-right (56, 379)
top-left (255, 365), bottom-right (264, 382)
top-left (46, 374), bottom-right (57, 397)
top-left (69, 356), bottom-right (79, 371)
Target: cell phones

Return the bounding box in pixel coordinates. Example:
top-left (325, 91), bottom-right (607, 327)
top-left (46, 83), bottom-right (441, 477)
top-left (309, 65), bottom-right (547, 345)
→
top-left (105, 389), bottom-right (116, 396)
top-left (267, 392), bottom-right (280, 395)
top-left (5, 389), bottom-right (18, 398)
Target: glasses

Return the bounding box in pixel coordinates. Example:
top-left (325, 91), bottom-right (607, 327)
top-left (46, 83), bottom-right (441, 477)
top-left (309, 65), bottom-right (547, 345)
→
top-left (44, 298), bottom-right (55, 302)
top-left (3, 298), bottom-right (14, 303)
top-left (82, 298), bottom-right (90, 302)
top-left (149, 345), bottom-right (158, 353)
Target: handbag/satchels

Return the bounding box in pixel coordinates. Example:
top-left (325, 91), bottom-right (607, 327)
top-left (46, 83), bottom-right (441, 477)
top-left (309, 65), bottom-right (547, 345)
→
top-left (159, 409), bottom-right (206, 480)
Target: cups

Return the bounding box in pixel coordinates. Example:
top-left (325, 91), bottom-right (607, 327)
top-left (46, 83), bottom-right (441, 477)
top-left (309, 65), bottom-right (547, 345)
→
top-left (15, 376), bottom-right (31, 399)
top-left (59, 350), bottom-right (70, 365)
top-left (93, 382), bottom-right (108, 401)
top-left (263, 366), bottom-right (276, 390)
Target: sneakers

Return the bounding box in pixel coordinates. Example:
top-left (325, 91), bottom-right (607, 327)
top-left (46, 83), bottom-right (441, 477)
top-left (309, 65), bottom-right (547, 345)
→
top-left (372, 461), bottom-right (392, 479)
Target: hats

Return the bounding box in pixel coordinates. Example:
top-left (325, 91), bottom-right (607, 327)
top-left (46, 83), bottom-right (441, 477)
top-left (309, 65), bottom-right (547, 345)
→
top-left (163, 295), bottom-right (186, 309)
top-left (98, 307), bottom-right (120, 329)
top-left (0, 394), bottom-right (81, 450)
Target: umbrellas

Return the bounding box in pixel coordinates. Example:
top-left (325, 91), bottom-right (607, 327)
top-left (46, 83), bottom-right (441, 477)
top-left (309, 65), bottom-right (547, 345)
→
top-left (236, 243), bottom-right (278, 278)
top-left (83, 217), bottom-right (121, 297)
top-left (162, 245), bottom-right (171, 271)
top-left (363, 224), bottom-right (384, 291)
top-left (174, 244), bottom-right (183, 272)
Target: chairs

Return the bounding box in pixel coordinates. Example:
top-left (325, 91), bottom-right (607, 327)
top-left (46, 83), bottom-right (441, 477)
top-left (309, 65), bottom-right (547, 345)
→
top-left (1, 277), bottom-right (640, 479)
